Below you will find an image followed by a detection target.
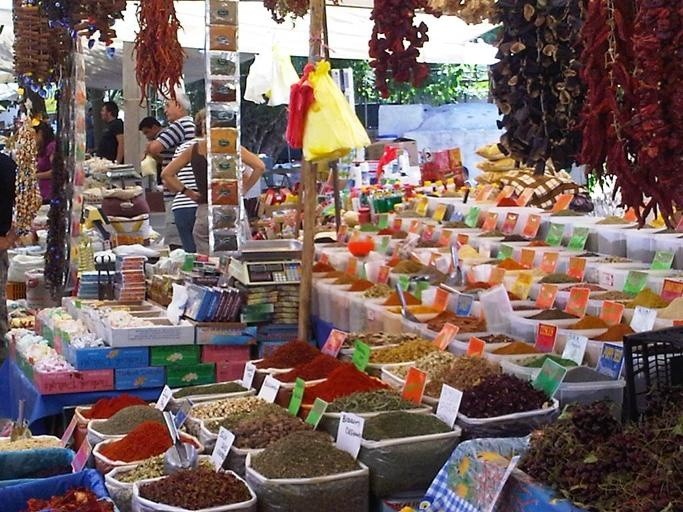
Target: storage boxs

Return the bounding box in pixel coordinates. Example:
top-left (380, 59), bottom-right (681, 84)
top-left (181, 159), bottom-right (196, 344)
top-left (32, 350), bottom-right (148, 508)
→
top-left (367, 137), bottom-right (418, 167)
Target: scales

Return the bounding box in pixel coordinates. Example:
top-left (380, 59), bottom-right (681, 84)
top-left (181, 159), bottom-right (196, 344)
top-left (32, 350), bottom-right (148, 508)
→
top-left (107, 164), bottom-right (141, 180)
top-left (227, 239), bottom-right (303, 288)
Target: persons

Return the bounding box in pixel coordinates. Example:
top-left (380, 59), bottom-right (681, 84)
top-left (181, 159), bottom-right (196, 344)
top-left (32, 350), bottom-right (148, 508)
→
top-left (85, 93), bottom-right (265, 259)
top-left (0, 153), bottom-right (43, 365)
top-left (35, 120), bottom-right (60, 206)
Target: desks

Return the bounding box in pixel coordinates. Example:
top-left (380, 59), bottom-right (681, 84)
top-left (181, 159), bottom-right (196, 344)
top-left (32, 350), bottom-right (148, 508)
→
top-left (0, 355), bottom-right (163, 435)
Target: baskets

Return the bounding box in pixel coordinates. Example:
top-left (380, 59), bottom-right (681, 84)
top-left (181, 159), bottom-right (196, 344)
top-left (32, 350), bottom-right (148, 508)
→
top-left (623, 326), bottom-right (683, 420)
